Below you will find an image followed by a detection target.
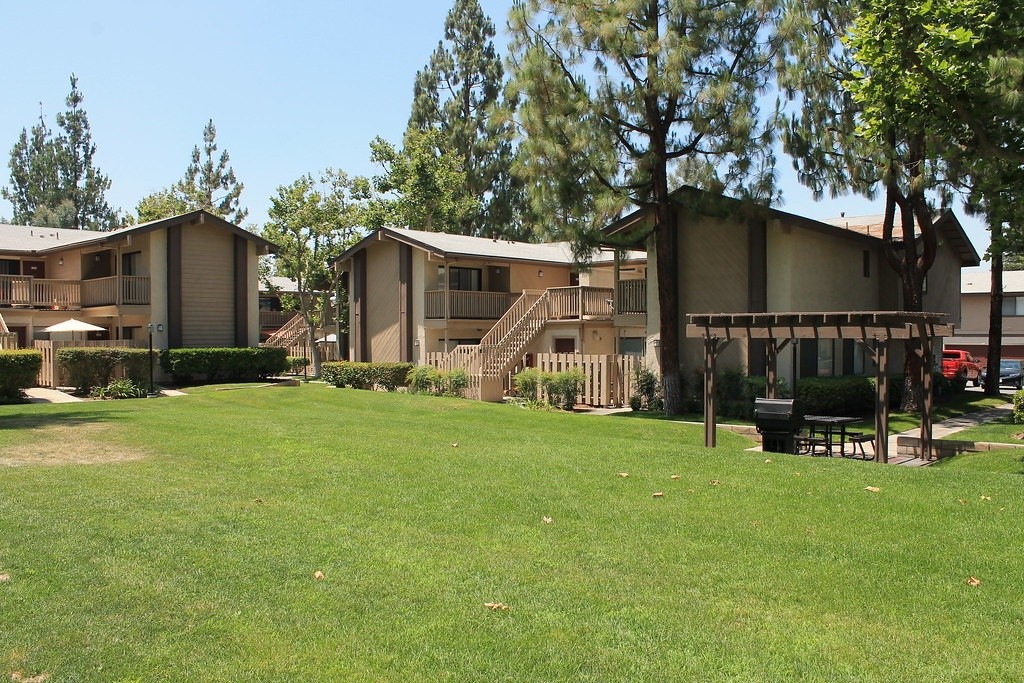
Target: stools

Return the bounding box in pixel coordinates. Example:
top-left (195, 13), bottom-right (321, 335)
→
top-left (811, 430), bottom-right (863, 455)
top-left (798, 437), bottom-right (830, 457)
top-left (849, 434), bottom-right (876, 461)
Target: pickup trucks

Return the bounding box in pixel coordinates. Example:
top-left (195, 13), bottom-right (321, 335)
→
top-left (941, 350), bottom-right (981, 386)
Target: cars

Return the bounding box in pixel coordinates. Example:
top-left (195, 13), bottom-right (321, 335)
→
top-left (978, 359), bottom-right (1024, 389)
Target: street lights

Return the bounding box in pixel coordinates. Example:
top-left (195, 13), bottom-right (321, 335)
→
top-left (144, 323), bottom-right (164, 397)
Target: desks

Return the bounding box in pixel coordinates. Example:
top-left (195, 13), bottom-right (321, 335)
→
top-left (801, 415), bottom-right (864, 457)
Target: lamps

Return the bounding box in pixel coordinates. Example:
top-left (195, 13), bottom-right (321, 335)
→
top-left (147, 324), bottom-right (157, 396)
